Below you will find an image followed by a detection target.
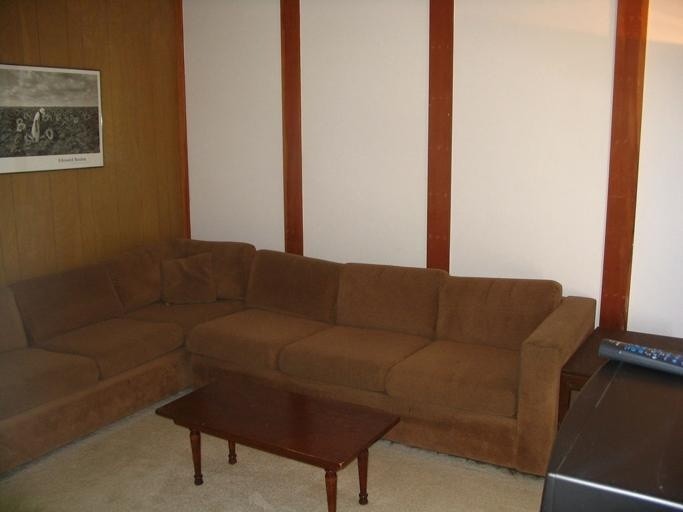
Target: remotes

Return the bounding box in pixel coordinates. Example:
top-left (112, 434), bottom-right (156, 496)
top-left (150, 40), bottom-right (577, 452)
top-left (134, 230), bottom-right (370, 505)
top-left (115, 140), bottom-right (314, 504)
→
top-left (597, 338), bottom-right (683, 376)
